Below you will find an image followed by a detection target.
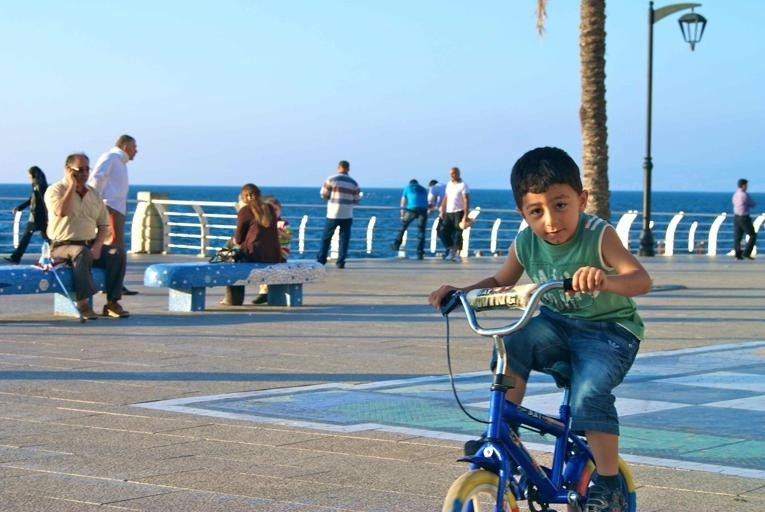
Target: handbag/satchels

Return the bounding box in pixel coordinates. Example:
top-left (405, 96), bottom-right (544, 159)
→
top-left (209, 248), bottom-right (241, 263)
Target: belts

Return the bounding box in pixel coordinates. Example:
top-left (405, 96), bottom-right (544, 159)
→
top-left (52, 240), bottom-right (97, 247)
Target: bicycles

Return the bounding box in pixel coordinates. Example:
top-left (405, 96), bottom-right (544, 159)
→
top-left (430, 278), bottom-right (638, 511)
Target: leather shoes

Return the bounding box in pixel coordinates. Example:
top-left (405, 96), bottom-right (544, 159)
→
top-left (252, 294), bottom-right (267, 304)
top-left (3, 256), bottom-right (18, 264)
top-left (120, 286), bottom-right (137, 295)
top-left (742, 254), bottom-right (753, 260)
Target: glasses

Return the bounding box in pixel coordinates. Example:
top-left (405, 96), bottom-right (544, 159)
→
top-left (70, 167), bottom-right (88, 172)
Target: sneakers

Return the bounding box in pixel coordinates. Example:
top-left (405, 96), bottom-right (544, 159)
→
top-left (582, 475), bottom-right (629, 511)
top-left (75, 303), bottom-right (98, 320)
top-left (444, 251), bottom-right (454, 260)
top-left (103, 303), bottom-right (129, 318)
top-left (390, 244), bottom-right (399, 251)
top-left (417, 254), bottom-right (423, 259)
top-left (452, 256), bottom-right (461, 263)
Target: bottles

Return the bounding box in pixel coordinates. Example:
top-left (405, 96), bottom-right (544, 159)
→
top-left (41, 240), bottom-right (51, 270)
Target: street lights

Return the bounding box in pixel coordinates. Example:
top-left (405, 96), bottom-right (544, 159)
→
top-left (636, 2), bottom-right (708, 257)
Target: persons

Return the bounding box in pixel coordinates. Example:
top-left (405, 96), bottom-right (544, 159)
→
top-left (2, 165), bottom-right (50, 265)
top-left (317, 159), bottom-right (361, 269)
top-left (252, 194), bottom-right (293, 305)
top-left (731, 178), bottom-right (757, 261)
top-left (85, 134), bottom-right (139, 295)
top-left (427, 145), bottom-right (655, 512)
top-left (390, 167), bottom-right (471, 263)
top-left (43, 152), bottom-right (131, 320)
top-left (217, 182), bottom-right (281, 305)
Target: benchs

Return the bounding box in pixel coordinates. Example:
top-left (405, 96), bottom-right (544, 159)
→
top-left (0, 264), bottom-right (107, 322)
top-left (143, 260), bottom-right (326, 312)
